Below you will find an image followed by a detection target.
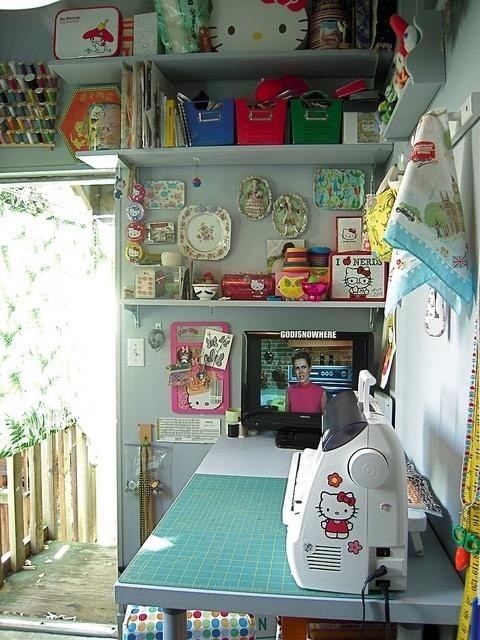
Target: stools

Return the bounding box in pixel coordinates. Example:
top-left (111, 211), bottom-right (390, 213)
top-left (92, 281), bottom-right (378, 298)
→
top-left (119, 599), bottom-right (259, 640)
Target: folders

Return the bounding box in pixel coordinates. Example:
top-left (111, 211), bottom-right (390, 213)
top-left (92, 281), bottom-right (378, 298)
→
top-left (143, 59), bottom-right (175, 129)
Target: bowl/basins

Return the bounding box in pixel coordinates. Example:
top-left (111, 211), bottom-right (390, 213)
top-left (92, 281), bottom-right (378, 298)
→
top-left (191, 284), bottom-right (220, 300)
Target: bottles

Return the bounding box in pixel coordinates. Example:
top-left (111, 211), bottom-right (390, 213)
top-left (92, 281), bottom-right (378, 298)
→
top-left (329, 355), bottom-right (334, 366)
top-left (320, 353), bottom-right (325, 365)
top-left (225, 406), bottom-right (244, 439)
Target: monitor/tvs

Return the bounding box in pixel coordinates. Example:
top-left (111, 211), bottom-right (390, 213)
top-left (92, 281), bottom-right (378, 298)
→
top-left (242, 331), bottom-right (374, 449)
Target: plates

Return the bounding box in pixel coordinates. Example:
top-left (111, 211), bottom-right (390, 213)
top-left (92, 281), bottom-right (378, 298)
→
top-left (288, 247), bottom-right (329, 268)
top-left (177, 206), bottom-right (232, 261)
top-left (312, 167), bottom-right (367, 211)
top-left (142, 180), bottom-right (184, 209)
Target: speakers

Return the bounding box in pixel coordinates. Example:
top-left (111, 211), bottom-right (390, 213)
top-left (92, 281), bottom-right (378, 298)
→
top-left (286, 368), bottom-right (408, 595)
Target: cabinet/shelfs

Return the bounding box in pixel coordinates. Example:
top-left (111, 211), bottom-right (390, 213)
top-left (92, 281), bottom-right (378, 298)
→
top-left (46, 42), bottom-right (395, 312)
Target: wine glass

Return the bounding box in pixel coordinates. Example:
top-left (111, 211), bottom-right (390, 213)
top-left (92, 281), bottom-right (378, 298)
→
top-left (299, 281), bottom-right (327, 300)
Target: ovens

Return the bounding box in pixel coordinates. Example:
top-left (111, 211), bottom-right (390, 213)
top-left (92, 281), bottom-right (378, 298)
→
top-left (290, 383), bottom-right (352, 403)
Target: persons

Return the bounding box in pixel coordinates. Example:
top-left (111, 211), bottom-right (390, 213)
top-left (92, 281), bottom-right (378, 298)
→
top-left (285, 352), bottom-right (326, 415)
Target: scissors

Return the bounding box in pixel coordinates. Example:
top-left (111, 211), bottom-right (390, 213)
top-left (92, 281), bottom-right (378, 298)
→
top-left (452, 526), bottom-right (479, 570)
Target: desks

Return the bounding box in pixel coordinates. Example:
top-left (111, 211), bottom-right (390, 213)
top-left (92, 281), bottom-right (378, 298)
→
top-left (110, 433), bottom-right (469, 639)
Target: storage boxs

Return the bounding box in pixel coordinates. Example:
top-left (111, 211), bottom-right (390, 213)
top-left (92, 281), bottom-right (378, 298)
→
top-left (287, 93), bottom-right (345, 146)
top-left (182, 96), bottom-right (237, 147)
top-left (236, 98), bottom-right (288, 147)
top-left (344, 100), bottom-right (387, 142)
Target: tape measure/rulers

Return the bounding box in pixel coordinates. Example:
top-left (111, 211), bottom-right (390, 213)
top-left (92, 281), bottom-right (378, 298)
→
top-left (459, 350), bottom-right (479, 639)
